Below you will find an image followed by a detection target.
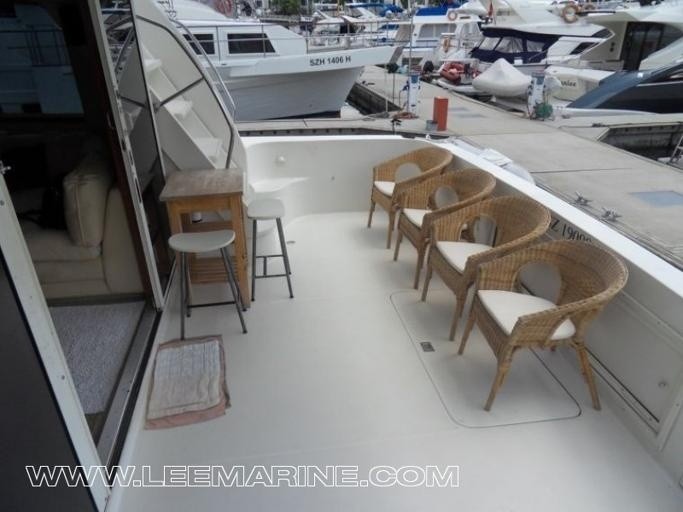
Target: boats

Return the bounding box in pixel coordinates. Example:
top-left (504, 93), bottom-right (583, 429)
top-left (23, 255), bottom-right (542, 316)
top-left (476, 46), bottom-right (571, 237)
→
top-left (156, 0), bottom-right (413, 118)
top-left (301, 0), bottom-right (683, 148)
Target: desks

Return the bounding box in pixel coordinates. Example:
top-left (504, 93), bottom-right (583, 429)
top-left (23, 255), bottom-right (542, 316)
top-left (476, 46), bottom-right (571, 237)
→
top-left (159, 168), bottom-right (250, 308)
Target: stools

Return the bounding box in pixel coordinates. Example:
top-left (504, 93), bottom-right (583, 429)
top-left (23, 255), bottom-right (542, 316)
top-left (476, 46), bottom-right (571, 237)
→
top-left (168, 230), bottom-right (247, 340)
top-left (247, 199), bottom-right (295, 301)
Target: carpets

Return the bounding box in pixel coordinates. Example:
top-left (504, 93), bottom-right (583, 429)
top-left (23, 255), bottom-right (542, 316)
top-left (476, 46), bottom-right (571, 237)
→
top-left (142, 334), bottom-right (234, 429)
top-left (49, 301), bottom-right (146, 414)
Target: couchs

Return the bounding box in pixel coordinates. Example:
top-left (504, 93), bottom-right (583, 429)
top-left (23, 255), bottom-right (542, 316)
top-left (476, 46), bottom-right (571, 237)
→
top-left (0, 131), bottom-right (145, 298)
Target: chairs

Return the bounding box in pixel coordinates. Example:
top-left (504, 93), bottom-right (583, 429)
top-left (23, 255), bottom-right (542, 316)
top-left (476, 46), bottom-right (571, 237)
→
top-left (422, 195), bottom-right (552, 341)
top-left (367, 148), bottom-right (454, 248)
top-left (458, 239), bottom-right (629, 412)
top-left (393, 168), bottom-right (496, 288)
